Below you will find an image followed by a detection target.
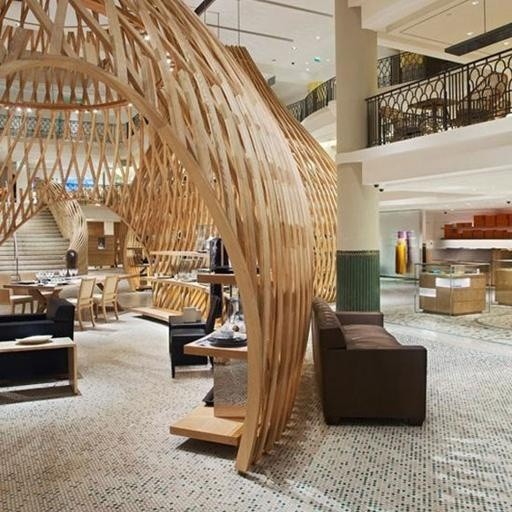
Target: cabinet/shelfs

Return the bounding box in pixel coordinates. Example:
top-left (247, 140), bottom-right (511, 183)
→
top-left (127, 250), bottom-right (273, 447)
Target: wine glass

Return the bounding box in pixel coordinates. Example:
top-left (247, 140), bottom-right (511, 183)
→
top-left (33, 269), bottom-right (79, 285)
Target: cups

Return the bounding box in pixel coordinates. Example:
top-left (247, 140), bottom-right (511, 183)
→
top-left (220, 330), bottom-right (235, 338)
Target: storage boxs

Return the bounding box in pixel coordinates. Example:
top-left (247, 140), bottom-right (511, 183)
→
top-left (444, 214), bottom-right (512, 239)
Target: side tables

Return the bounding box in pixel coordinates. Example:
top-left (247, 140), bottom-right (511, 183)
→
top-left (0, 337), bottom-right (78, 394)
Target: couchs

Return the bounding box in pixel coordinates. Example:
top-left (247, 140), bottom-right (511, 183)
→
top-left (309, 296), bottom-right (427, 426)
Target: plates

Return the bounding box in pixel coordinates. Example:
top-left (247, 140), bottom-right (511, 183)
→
top-left (157, 274), bottom-right (196, 281)
top-left (206, 334), bottom-right (247, 348)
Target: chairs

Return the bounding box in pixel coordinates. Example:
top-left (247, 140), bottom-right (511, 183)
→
top-left (456, 72), bottom-right (508, 128)
top-left (378, 106), bottom-right (422, 143)
top-left (0, 296), bottom-right (76, 381)
top-left (65, 278), bottom-right (97, 329)
top-left (413, 261), bottom-right (492, 317)
top-left (494, 259), bottom-right (512, 306)
top-left (88, 274), bottom-right (121, 322)
top-left (8, 273), bottom-right (34, 315)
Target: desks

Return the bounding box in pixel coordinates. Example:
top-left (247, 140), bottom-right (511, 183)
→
top-left (408, 98), bottom-right (457, 133)
